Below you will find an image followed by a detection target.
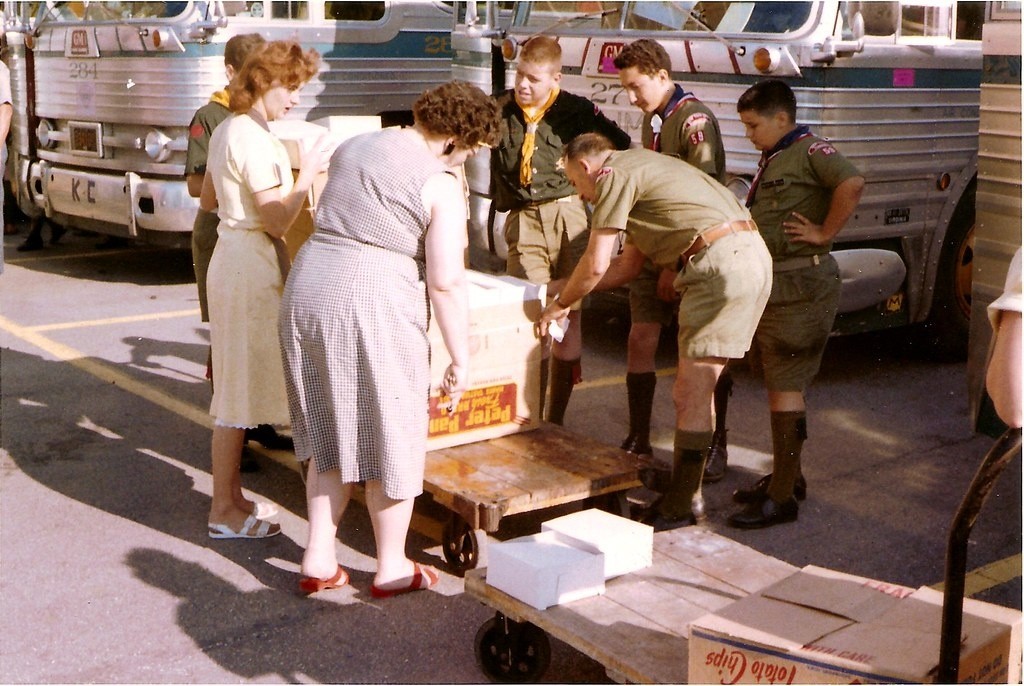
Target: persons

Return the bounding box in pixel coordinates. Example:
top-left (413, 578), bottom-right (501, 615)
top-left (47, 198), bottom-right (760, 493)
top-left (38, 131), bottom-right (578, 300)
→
top-left (185, 36), bottom-right (865, 539)
top-left (277, 82), bottom-right (499, 597)
top-left (986, 242), bottom-right (1022, 429)
top-left (0, 15), bottom-right (13, 275)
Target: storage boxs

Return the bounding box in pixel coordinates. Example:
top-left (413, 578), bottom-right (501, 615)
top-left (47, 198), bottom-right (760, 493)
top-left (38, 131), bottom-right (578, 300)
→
top-left (688, 564), bottom-right (1022, 685)
top-left (270, 115), bottom-right (548, 453)
top-left (485, 507), bottom-right (654, 611)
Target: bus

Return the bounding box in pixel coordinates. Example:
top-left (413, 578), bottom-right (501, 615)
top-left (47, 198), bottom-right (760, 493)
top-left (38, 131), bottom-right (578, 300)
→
top-left (1, 0), bottom-right (1021, 362)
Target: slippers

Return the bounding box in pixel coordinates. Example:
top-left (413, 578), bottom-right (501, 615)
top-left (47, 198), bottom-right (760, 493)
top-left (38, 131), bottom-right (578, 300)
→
top-left (299, 562), bottom-right (351, 594)
top-left (369, 561), bottom-right (440, 599)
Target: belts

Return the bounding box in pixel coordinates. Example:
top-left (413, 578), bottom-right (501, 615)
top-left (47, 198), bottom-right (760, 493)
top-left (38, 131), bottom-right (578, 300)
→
top-left (674, 218), bottom-right (758, 271)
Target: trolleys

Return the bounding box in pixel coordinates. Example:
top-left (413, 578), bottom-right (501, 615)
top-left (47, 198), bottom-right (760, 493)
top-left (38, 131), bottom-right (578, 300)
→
top-left (240, 260), bottom-right (666, 574)
top-left (461, 426), bottom-right (1022, 684)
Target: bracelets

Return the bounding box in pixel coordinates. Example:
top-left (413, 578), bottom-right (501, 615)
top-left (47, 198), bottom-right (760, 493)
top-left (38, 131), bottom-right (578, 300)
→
top-left (555, 296), bottom-right (569, 310)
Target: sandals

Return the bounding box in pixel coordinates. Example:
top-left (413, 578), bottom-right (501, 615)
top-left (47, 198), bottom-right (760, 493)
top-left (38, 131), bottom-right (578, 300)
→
top-left (206, 510), bottom-right (280, 539)
top-left (254, 499), bottom-right (280, 519)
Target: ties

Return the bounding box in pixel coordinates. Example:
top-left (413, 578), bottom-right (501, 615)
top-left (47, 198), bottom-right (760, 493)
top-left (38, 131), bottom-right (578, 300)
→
top-left (515, 86), bottom-right (560, 186)
top-left (648, 81), bottom-right (700, 151)
top-left (746, 127), bottom-right (816, 209)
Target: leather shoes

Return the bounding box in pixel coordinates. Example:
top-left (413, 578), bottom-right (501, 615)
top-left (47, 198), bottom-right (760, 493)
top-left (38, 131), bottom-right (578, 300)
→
top-left (728, 496), bottom-right (799, 529)
top-left (733, 471), bottom-right (807, 502)
top-left (635, 500), bottom-right (694, 533)
top-left (700, 435), bottom-right (729, 482)
top-left (619, 439), bottom-right (654, 459)
